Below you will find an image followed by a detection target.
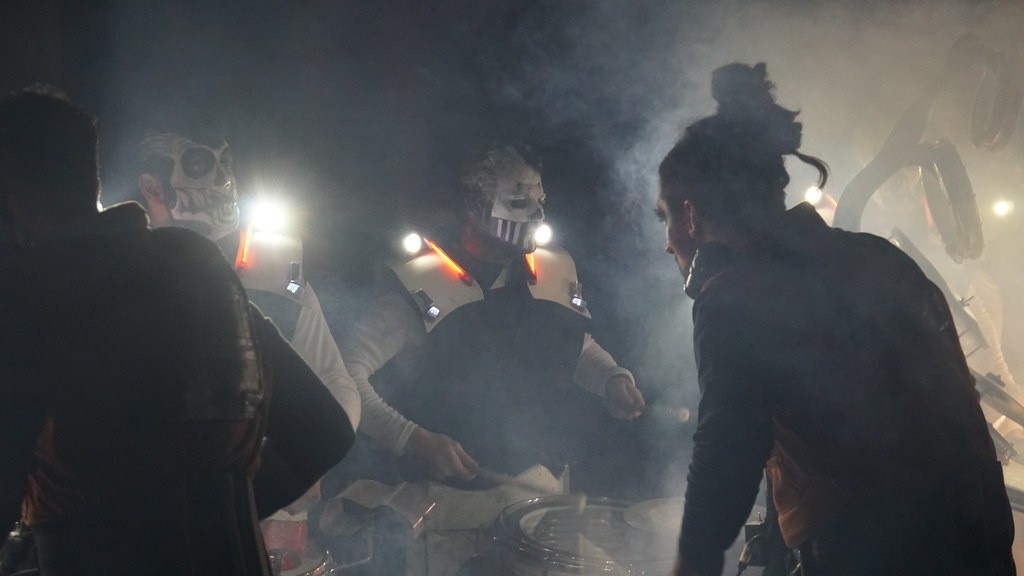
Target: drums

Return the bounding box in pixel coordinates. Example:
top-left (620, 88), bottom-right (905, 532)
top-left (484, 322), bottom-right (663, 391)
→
top-left (624, 496), bottom-right (766, 576)
top-left (490, 493), bottom-right (679, 576)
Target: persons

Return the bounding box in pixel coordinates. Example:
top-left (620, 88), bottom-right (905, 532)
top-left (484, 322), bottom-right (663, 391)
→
top-left (655, 61), bottom-right (1017, 576)
top-left (0, 92), bottom-right (356, 576)
top-left (132, 106), bottom-right (361, 576)
top-left (340, 142), bottom-right (644, 576)
